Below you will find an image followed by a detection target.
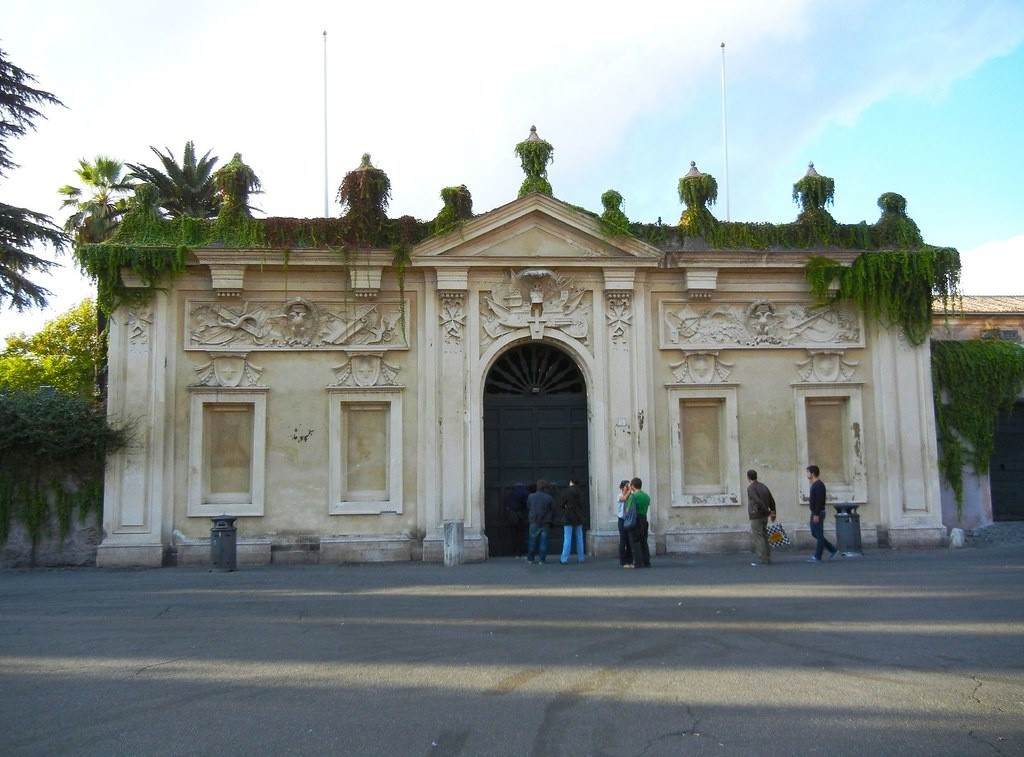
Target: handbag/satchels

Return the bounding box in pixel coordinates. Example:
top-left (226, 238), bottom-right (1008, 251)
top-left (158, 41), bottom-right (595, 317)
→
top-left (766, 519), bottom-right (790, 549)
top-left (623, 492), bottom-right (636, 530)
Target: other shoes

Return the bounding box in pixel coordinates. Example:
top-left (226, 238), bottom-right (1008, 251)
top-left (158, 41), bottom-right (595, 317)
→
top-left (538, 562), bottom-right (545, 566)
top-left (829, 550), bottom-right (839, 561)
top-left (528, 561), bottom-right (533, 564)
top-left (750, 563), bottom-right (757, 566)
top-left (623, 564), bottom-right (635, 568)
top-left (807, 556), bottom-right (821, 562)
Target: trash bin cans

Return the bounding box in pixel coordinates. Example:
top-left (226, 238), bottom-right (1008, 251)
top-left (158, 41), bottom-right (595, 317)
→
top-left (832, 502), bottom-right (863, 557)
top-left (208, 515), bottom-right (238, 572)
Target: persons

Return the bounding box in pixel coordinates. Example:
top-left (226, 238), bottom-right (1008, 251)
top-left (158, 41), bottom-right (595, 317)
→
top-left (555, 476), bottom-right (587, 565)
top-left (626, 477), bottom-right (651, 569)
top-left (746, 470), bottom-right (776, 567)
top-left (526, 480), bottom-right (554, 565)
top-left (806, 465), bottom-right (842, 563)
top-left (616, 480), bottom-right (634, 569)
top-left (506, 473), bottom-right (531, 559)
